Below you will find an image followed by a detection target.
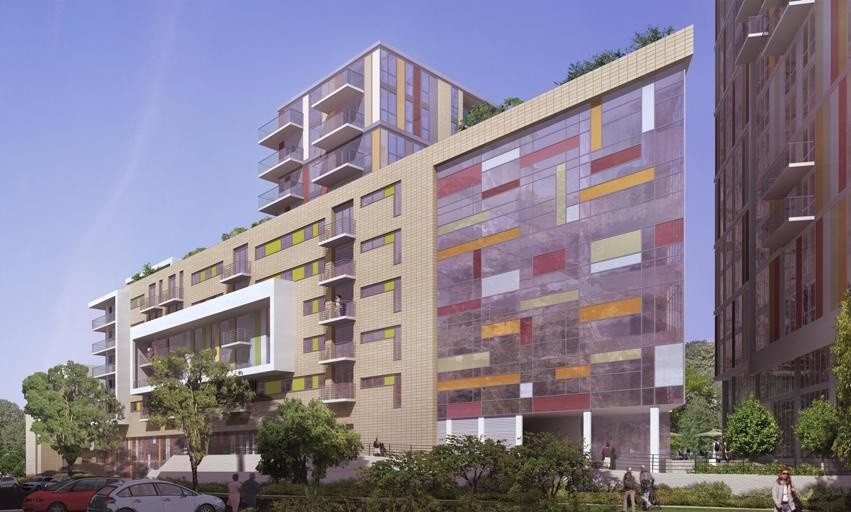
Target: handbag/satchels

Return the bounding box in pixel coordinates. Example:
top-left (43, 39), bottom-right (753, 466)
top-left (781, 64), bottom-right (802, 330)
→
top-left (602, 456), bottom-right (612, 468)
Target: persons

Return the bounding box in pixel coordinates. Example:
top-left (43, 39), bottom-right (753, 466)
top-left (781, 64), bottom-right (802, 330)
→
top-left (374, 437), bottom-right (382, 456)
top-left (335, 295), bottom-right (343, 316)
top-left (772, 466), bottom-right (798, 512)
top-left (227, 473), bottom-right (243, 511)
top-left (600, 441), bottom-right (616, 469)
top-left (722, 441), bottom-right (731, 463)
top-left (240, 472), bottom-right (261, 509)
top-left (639, 464), bottom-right (655, 510)
top-left (677, 446), bottom-right (689, 460)
top-left (715, 441), bottom-right (723, 463)
top-left (621, 466), bottom-right (637, 512)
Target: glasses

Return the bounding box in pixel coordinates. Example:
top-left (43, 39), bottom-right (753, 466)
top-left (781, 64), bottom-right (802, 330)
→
top-left (782, 472), bottom-right (790, 476)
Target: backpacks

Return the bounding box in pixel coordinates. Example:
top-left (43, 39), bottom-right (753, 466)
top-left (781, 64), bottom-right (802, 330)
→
top-left (792, 493), bottom-right (802, 511)
top-left (624, 478), bottom-right (633, 489)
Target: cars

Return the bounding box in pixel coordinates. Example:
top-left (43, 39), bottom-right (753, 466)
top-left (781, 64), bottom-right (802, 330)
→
top-left (0, 477), bottom-right (226, 512)
